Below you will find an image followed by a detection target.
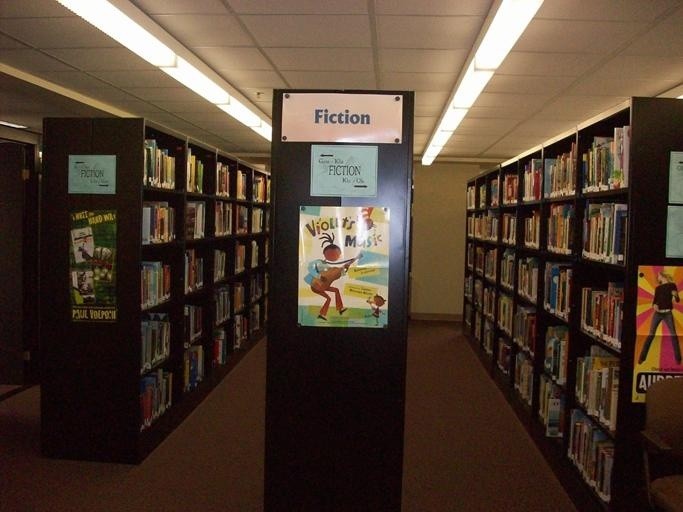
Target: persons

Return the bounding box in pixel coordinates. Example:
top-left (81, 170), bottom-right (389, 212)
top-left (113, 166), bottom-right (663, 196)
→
top-left (636, 271), bottom-right (681, 365)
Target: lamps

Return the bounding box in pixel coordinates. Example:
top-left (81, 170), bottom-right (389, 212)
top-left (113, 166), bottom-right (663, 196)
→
top-left (417, 0), bottom-right (544, 166)
top-left (55, 1), bottom-right (271, 144)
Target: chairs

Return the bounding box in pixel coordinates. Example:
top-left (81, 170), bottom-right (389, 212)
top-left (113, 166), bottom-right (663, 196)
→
top-left (637, 376), bottom-right (682, 512)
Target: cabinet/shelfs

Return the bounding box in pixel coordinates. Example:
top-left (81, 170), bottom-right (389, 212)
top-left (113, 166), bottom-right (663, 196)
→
top-left (462, 94), bottom-right (683, 512)
top-left (264, 88), bottom-right (415, 512)
top-left (42, 116), bottom-right (272, 466)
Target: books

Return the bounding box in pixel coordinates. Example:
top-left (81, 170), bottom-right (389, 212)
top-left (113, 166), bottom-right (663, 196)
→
top-left (465, 160), bottom-right (541, 405)
top-left (539, 144), bottom-right (576, 437)
top-left (566, 125), bottom-right (631, 500)
top-left (215, 162), bottom-right (270, 364)
top-left (184, 148), bottom-right (205, 392)
top-left (141, 140), bottom-right (175, 430)
